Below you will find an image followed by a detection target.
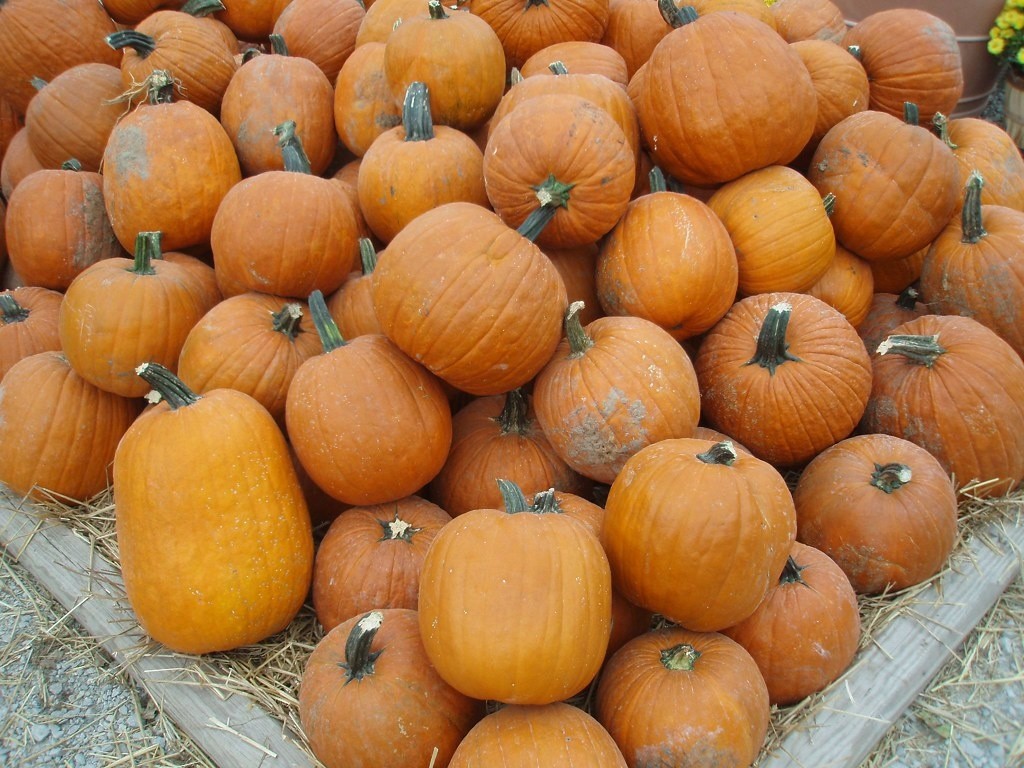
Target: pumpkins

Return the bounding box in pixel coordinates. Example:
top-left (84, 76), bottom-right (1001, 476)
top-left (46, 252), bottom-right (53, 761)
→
top-left (0, 0), bottom-right (1024, 768)
